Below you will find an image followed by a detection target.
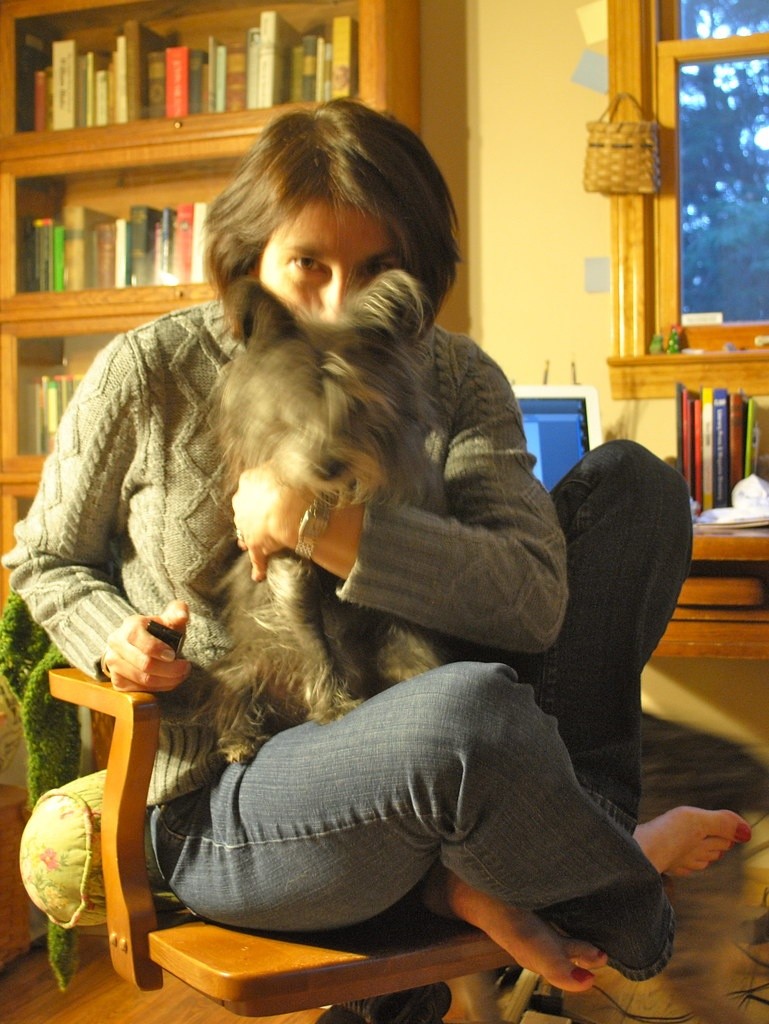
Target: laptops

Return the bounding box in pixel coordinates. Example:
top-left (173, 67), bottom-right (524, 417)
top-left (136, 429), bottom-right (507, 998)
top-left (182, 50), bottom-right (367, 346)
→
top-left (512, 386), bottom-right (603, 494)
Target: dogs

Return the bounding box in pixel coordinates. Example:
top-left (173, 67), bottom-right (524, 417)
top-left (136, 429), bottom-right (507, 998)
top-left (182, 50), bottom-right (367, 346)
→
top-left (183, 270), bottom-right (453, 767)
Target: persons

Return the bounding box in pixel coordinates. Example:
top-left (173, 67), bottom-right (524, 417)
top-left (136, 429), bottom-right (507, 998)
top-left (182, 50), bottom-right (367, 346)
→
top-left (14, 100), bottom-right (754, 994)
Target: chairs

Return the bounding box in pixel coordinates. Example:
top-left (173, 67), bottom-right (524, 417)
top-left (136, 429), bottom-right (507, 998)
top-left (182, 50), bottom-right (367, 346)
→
top-left (0, 525), bottom-right (533, 1024)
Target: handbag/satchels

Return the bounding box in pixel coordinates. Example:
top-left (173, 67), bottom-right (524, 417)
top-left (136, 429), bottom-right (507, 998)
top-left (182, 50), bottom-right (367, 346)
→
top-left (583, 93), bottom-right (662, 194)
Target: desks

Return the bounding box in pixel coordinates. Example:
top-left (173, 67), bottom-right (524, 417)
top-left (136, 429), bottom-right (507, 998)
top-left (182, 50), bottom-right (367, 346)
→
top-left (640, 525), bottom-right (768, 662)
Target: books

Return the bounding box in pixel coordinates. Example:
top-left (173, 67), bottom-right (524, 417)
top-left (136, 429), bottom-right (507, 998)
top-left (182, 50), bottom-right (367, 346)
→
top-left (666, 381), bottom-right (769, 524)
top-left (14, 10), bottom-right (358, 471)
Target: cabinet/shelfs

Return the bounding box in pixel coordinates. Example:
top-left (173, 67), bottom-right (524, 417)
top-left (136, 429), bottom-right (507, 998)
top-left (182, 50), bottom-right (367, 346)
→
top-left (0, 0), bottom-right (423, 628)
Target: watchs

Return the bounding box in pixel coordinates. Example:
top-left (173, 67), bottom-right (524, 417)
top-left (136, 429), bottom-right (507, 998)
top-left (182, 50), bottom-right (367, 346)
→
top-left (293, 489), bottom-right (337, 561)
